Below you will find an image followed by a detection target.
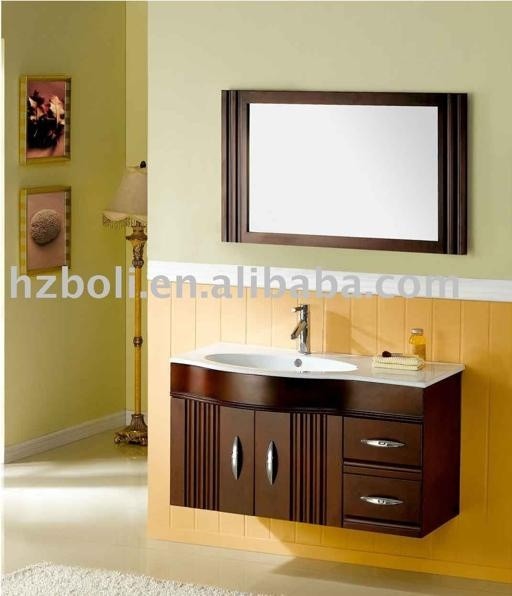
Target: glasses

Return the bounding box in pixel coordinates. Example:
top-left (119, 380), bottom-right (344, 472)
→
top-left (101, 159), bottom-right (148, 450)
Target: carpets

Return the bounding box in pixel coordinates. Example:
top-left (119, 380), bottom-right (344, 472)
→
top-left (2, 560), bottom-right (265, 596)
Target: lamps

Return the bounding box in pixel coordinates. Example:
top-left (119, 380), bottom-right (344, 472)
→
top-left (101, 159), bottom-right (148, 450)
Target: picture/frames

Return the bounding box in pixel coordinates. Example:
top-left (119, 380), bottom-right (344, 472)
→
top-left (18, 73), bottom-right (73, 276)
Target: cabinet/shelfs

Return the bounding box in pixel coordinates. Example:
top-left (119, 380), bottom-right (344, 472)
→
top-left (169, 395), bottom-right (460, 539)
top-left (408, 329), bottom-right (426, 362)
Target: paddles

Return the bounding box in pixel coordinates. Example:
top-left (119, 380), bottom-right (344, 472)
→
top-left (2, 560), bottom-right (265, 596)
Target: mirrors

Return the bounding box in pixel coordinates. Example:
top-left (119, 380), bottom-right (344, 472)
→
top-left (219, 88), bottom-right (468, 255)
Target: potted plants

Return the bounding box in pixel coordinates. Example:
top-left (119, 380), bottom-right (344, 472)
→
top-left (18, 73), bottom-right (73, 276)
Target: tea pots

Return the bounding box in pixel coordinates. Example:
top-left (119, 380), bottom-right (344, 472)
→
top-left (219, 88), bottom-right (468, 255)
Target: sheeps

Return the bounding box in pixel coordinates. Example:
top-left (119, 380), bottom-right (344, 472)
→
top-left (288, 304), bottom-right (310, 353)
